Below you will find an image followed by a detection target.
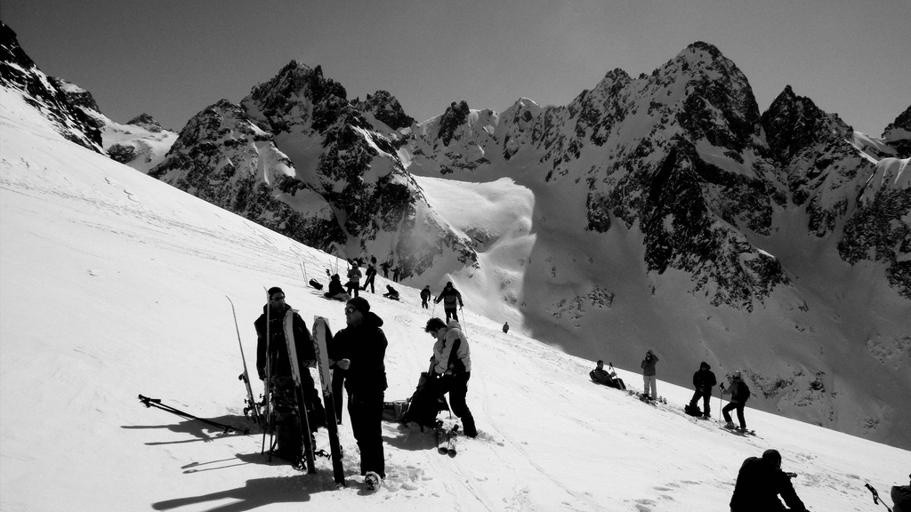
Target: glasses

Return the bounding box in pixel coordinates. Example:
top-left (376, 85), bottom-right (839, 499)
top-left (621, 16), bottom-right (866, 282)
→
top-left (275, 296), bottom-right (285, 300)
top-left (345, 306), bottom-right (355, 315)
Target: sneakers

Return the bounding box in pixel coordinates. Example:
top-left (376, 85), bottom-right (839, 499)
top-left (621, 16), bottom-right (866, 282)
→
top-left (365, 472), bottom-right (378, 488)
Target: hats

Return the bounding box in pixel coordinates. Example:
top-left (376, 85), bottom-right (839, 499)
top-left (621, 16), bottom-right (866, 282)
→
top-left (347, 297), bottom-right (370, 316)
top-left (268, 287), bottom-right (285, 300)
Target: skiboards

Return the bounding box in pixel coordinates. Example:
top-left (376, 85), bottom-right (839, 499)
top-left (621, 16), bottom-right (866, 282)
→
top-left (226, 285), bottom-right (346, 487)
top-left (589, 379), bottom-right (757, 437)
top-left (436, 419), bottom-right (459, 457)
top-left (299, 256), bottom-right (349, 288)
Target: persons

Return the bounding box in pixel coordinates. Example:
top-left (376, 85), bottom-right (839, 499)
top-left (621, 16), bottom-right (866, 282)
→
top-left (720, 370), bottom-right (750, 432)
top-left (426, 318), bottom-right (479, 437)
top-left (594, 360), bottom-right (626, 390)
top-left (421, 282), bottom-right (463, 324)
top-left (503, 322), bottom-right (509, 333)
top-left (730, 449), bottom-right (810, 512)
top-left (328, 253), bottom-right (400, 301)
top-left (690, 362), bottom-right (717, 417)
top-left (255, 288), bottom-right (323, 470)
top-left (334, 297), bottom-right (387, 479)
top-left (641, 352), bottom-right (659, 400)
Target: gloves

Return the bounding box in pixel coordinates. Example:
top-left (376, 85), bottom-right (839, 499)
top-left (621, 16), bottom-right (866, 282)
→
top-left (335, 358), bottom-right (351, 370)
top-left (303, 361), bottom-right (315, 368)
